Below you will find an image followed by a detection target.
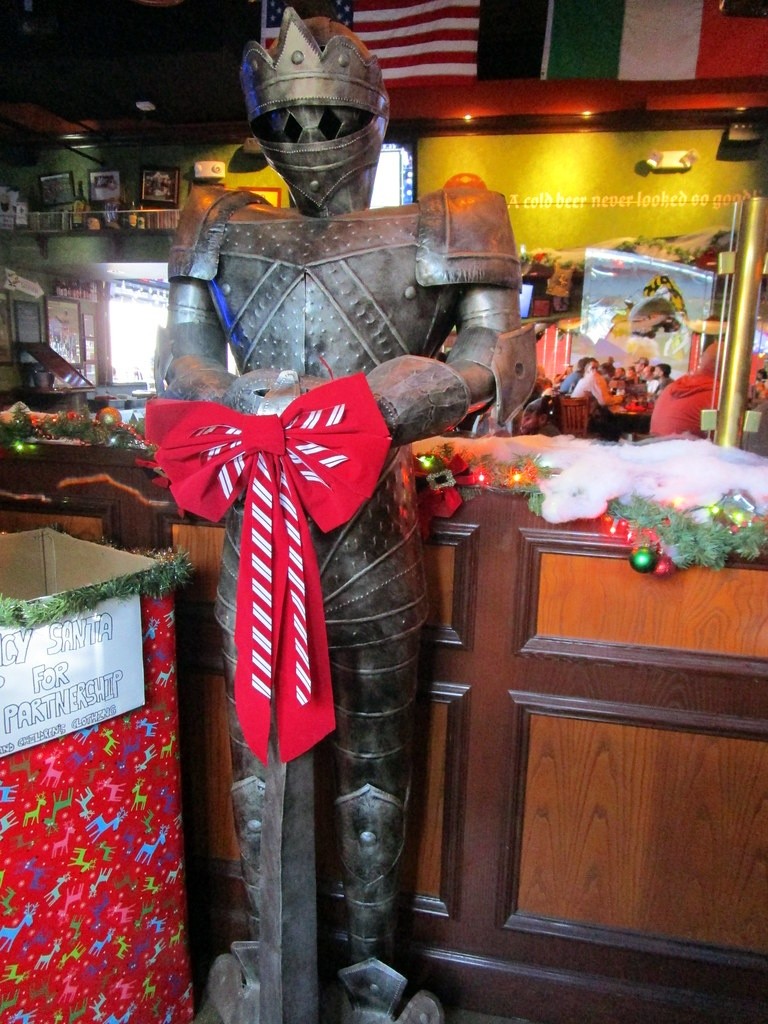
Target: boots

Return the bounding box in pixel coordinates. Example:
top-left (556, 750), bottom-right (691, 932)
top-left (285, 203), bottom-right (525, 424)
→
top-left (205, 776), bottom-right (445, 1024)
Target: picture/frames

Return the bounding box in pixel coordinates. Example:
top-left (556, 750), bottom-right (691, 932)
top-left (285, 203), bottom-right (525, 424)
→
top-left (87, 167), bottom-right (127, 206)
top-left (38, 169), bottom-right (76, 206)
top-left (136, 164), bottom-right (181, 210)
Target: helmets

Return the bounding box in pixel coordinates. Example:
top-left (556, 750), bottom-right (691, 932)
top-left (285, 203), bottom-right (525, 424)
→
top-left (242, 9), bottom-right (389, 209)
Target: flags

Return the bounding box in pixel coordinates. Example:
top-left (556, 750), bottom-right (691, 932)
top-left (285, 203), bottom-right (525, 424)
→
top-left (256, 1), bottom-right (485, 86)
top-left (538, 0), bottom-right (768, 84)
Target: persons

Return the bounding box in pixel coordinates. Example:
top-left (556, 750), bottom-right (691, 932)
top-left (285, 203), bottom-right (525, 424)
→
top-left (521, 347), bottom-right (678, 441)
top-left (156, 5), bottom-right (529, 1020)
top-left (647, 338), bottom-right (729, 443)
top-left (748, 369), bottom-right (768, 407)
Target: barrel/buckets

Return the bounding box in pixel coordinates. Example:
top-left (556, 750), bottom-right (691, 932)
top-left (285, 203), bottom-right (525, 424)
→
top-left (33, 369), bottom-right (56, 388)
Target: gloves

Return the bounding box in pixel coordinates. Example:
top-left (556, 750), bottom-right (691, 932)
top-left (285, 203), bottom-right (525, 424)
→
top-left (359, 355), bottom-right (470, 445)
top-left (162, 368), bottom-right (301, 417)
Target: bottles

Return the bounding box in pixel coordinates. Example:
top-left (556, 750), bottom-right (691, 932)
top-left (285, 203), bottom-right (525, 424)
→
top-left (56, 277), bottom-right (98, 301)
